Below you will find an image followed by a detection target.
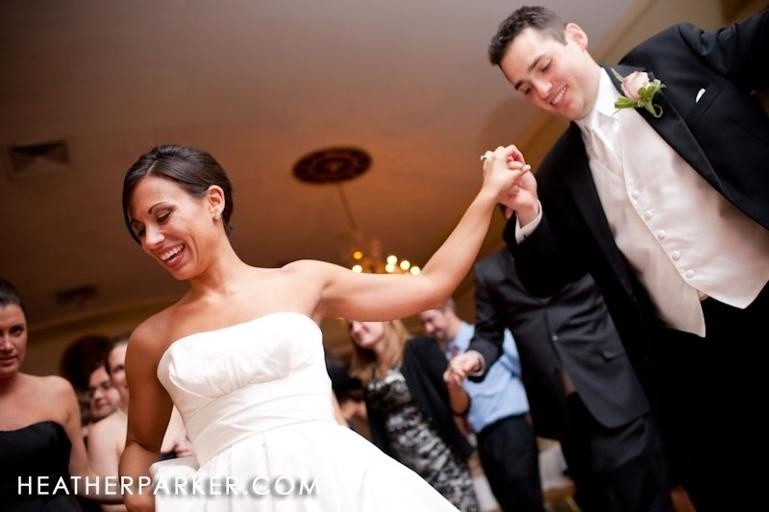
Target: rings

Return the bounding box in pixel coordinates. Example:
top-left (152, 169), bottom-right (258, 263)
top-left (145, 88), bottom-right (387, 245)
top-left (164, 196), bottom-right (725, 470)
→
top-left (480, 154), bottom-right (487, 162)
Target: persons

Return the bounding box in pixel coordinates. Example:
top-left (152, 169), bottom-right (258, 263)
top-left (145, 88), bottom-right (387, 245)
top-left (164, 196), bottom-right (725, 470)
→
top-left (341, 316), bottom-right (472, 512)
top-left (448, 202), bottom-right (680, 511)
top-left (0, 279), bottom-right (125, 511)
top-left (79, 363), bottom-right (122, 432)
top-left (84, 333), bottom-right (193, 510)
top-left (421, 295), bottom-right (547, 510)
top-left (476, 0), bottom-right (768, 510)
top-left (118, 140), bottom-right (533, 511)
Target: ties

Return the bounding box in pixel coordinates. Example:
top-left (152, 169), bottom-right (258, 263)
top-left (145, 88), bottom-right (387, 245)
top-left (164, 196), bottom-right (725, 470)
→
top-left (581, 109), bottom-right (622, 179)
